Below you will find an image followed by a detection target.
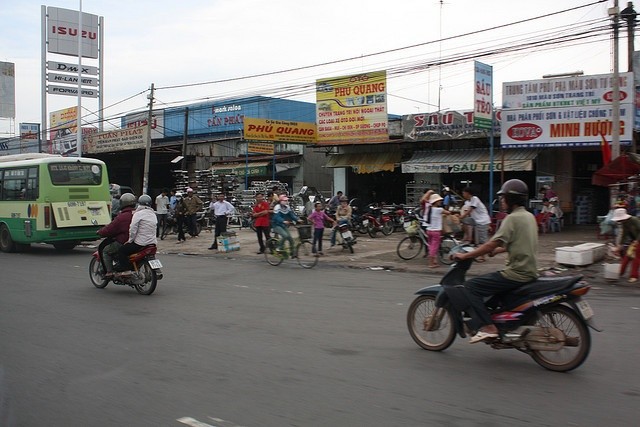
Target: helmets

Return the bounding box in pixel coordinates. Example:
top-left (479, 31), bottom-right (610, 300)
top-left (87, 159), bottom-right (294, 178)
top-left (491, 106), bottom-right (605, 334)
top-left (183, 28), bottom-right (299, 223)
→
top-left (139, 194), bottom-right (153, 206)
top-left (496, 179), bottom-right (528, 195)
top-left (118, 193), bottom-right (136, 210)
top-left (187, 188), bottom-right (194, 195)
top-left (175, 192), bottom-right (183, 197)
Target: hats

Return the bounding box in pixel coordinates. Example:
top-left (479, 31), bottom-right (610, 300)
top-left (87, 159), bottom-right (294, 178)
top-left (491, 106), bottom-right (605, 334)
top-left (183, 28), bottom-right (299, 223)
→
top-left (429, 193), bottom-right (443, 203)
top-left (279, 194), bottom-right (290, 201)
top-left (340, 195), bottom-right (349, 202)
top-left (610, 208), bottom-right (631, 222)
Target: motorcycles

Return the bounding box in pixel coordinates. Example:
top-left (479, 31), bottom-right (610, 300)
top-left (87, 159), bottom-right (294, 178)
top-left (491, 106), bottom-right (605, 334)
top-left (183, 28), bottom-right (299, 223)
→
top-left (89, 218), bottom-right (163, 294)
top-left (326, 196), bottom-right (358, 253)
top-left (407, 241), bottom-right (605, 371)
top-left (354, 201), bottom-right (407, 238)
top-left (160, 210), bottom-right (205, 239)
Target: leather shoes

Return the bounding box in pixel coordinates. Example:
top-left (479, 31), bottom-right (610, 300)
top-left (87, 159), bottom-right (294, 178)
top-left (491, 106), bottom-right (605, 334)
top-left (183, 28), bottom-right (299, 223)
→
top-left (256, 250), bottom-right (263, 254)
top-left (208, 245), bottom-right (216, 249)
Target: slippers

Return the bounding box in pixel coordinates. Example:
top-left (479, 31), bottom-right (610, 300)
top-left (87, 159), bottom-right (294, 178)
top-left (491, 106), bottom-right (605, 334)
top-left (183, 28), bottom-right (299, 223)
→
top-left (117, 271), bottom-right (131, 277)
top-left (104, 271), bottom-right (115, 277)
top-left (469, 330), bottom-right (498, 343)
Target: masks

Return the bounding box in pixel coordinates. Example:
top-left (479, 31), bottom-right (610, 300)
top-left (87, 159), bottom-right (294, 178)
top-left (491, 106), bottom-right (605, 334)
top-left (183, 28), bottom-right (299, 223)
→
top-left (281, 202), bottom-right (287, 205)
top-left (176, 197), bottom-right (181, 201)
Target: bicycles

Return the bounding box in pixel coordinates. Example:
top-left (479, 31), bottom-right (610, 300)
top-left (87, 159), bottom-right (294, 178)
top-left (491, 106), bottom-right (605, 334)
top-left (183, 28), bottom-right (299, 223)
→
top-left (265, 220), bottom-right (318, 269)
top-left (397, 210), bottom-right (458, 264)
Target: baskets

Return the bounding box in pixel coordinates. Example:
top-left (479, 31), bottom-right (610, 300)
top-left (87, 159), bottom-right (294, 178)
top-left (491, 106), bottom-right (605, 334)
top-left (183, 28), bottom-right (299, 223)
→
top-left (299, 225), bottom-right (311, 238)
top-left (403, 220), bottom-right (420, 235)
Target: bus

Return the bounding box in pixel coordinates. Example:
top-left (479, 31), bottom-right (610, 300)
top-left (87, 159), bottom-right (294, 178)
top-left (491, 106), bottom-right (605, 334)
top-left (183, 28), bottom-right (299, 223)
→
top-left (0, 152), bottom-right (112, 251)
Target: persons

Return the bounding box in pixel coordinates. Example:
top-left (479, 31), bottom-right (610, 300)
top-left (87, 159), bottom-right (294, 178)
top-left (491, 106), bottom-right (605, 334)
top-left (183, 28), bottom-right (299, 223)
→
top-left (306, 201), bottom-right (335, 257)
top-left (456, 189), bottom-right (491, 261)
top-left (327, 196), bottom-right (352, 250)
top-left (270, 194), bottom-right (303, 259)
top-left (167, 189), bottom-right (178, 235)
top-left (208, 193), bottom-right (235, 249)
top-left (442, 187), bottom-right (458, 215)
top-left (20, 183), bottom-right (26, 200)
top-left (97, 192), bottom-right (138, 278)
top-left (327, 191), bottom-right (343, 227)
top-left (155, 188), bottom-right (170, 238)
top-left (541, 199), bottom-right (558, 233)
top-left (252, 193), bottom-right (272, 254)
top-left (539, 187), bottom-right (560, 209)
top-left (420, 187), bottom-right (435, 208)
top-left (611, 209), bottom-right (640, 282)
top-left (174, 192), bottom-right (187, 244)
top-left (183, 188), bottom-right (203, 236)
top-left (449, 179), bottom-right (539, 344)
top-left (613, 196), bottom-right (629, 247)
top-left (267, 192), bottom-right (280, 239)
top-left (117, 195), bottom-right (158, 278)
top-left (422, 194), bottom-right (451, 268)
top-left (460, 199), bottom-right (473, 244)
top-left (112, 195), bottom-right (120, 221)
